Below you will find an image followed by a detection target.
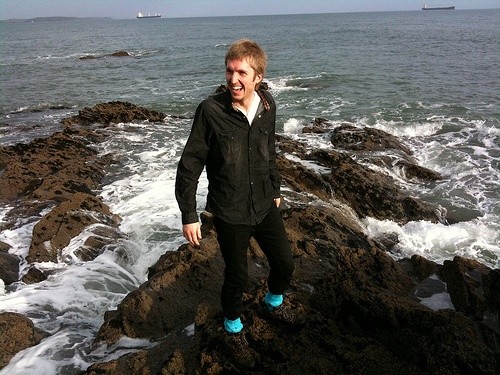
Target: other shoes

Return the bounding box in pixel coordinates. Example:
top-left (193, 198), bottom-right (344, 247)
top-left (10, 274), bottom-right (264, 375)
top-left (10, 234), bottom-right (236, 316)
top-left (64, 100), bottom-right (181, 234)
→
top-left (265, 289), bottom-right (284, 310)
top-left (224, 317), bottom-right (244, 334)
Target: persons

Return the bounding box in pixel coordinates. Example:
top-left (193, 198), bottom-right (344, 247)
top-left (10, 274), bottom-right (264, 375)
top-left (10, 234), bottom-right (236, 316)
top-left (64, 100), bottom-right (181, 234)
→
top-left (174, 39), bottom-right (299, 370)
